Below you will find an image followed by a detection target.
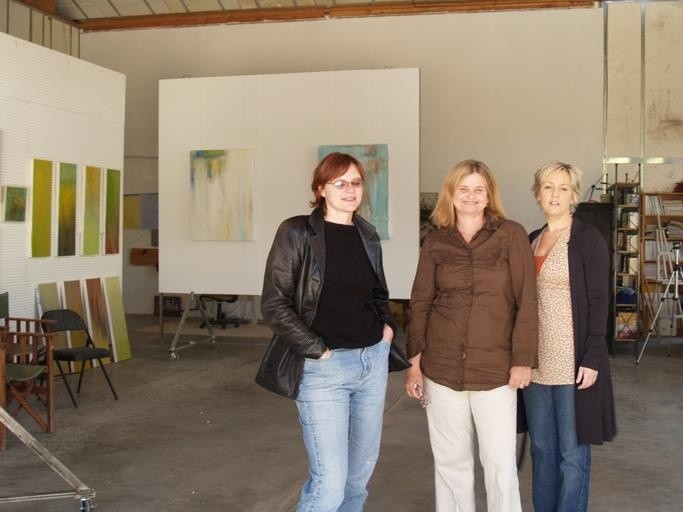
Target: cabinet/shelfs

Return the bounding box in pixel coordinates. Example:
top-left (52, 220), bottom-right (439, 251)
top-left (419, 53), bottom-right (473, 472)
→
top-left (603, 161), bottom-right (641, 358)
top-left (639, 191), bottom-right (683, 341)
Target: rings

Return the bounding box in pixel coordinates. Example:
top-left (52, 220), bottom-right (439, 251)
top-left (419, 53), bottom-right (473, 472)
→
top-left (518, 383), bottom-right (527, 388)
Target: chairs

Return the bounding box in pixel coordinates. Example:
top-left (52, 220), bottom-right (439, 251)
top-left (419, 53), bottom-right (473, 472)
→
top-left (40, 307), bottom-right (119, 409)
top-left (0, 314), bottom-right (58, 451)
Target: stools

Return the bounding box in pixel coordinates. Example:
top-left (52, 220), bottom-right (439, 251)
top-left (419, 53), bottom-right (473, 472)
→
top-left (196, 293), bottom-right (240, 330)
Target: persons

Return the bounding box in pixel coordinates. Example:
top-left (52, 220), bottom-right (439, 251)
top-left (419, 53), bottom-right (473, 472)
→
top-left (254, 153), bottom-right (412, 511)
top-left (516, 162), bottom-right (617, 511)
top-left (406, 159), bottom-right (538, 511)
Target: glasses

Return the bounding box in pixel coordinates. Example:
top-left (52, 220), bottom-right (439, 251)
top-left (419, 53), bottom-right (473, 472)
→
top-left (327, 178), bottom-right (363, 187)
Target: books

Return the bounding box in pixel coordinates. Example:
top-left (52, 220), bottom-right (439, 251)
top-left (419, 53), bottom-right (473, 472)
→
top-left (616, 192), bottom-right (681, 340)
